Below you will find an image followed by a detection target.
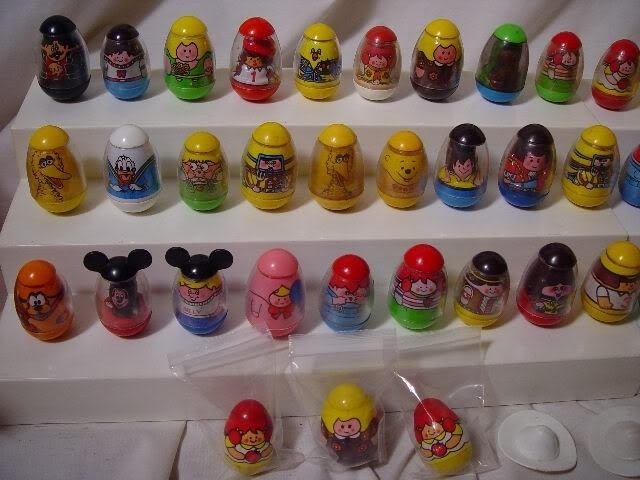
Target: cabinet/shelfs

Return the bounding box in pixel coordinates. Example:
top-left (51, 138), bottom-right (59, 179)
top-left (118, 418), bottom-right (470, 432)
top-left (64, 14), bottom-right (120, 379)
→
top-left (0, 61), bottom-right (640, 424)
